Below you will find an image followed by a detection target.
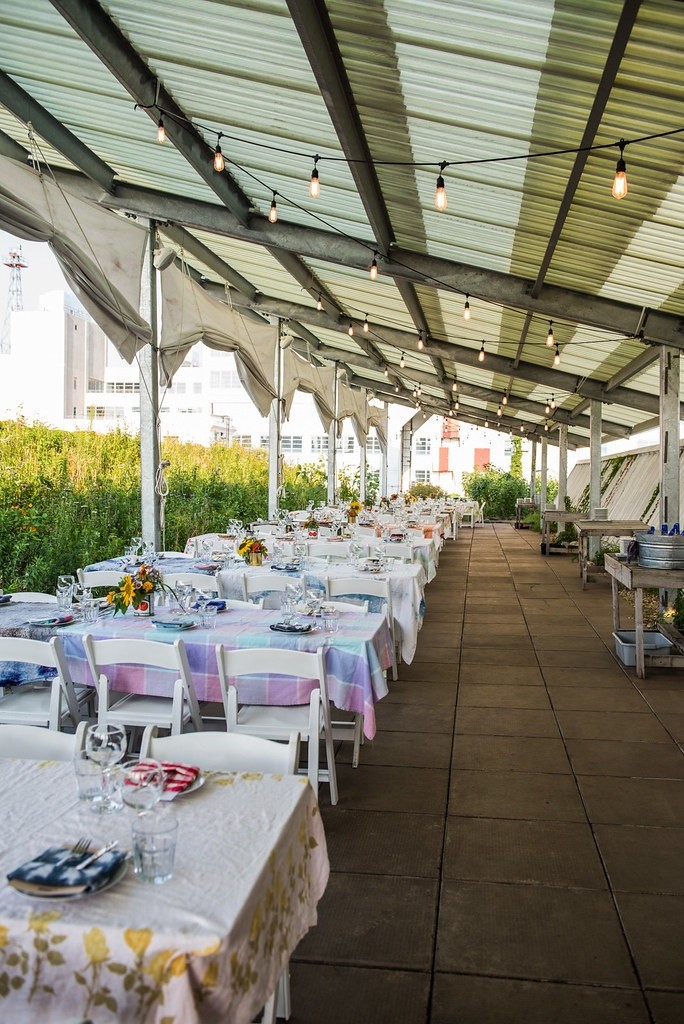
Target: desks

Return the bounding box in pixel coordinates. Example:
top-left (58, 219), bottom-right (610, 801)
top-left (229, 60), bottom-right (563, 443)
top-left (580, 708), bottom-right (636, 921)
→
top-left (76, 548), bottom-right (429, 666)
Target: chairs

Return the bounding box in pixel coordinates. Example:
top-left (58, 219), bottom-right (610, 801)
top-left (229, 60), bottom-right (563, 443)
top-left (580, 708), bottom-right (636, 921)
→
top-left (0, 497), bottom-right (486, 1024)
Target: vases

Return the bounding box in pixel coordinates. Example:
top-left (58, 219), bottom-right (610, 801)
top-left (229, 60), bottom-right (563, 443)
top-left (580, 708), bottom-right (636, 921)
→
top-left (248, 553), bottom-right (262, 566)
top-left (134, 589), bottom-right (156, 617)
top-left (348, 516), bottom-right (356, 523)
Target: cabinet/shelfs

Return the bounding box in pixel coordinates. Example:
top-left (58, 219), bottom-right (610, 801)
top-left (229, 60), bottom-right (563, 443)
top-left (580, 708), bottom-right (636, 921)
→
top-left (604, 553), bottom-right (684, 678)
top-left (573, 520), bottom-right (651, 591)
top-left (514, 504), bottom-right (537, 529)
top-left (541, 511), bottom-right (588, 557)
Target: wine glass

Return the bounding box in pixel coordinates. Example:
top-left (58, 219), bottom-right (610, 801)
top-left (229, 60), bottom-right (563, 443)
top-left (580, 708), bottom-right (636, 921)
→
top-left (72, 582), bottom-right (91, 621)
top-left (57, 575), bottom-right (75, 604)
top-left (174, 580), bottom-right (192, 614)
top-left (285, 582), bottom-right (303, 619)
top-left (119, 758), bottom-right (165, 858)
top-left (85, 723), bottom-right (128, 814)
top-left (195, 586), bottom-right (212, 612)
top-left (305, 588), bottom-right (324, 630)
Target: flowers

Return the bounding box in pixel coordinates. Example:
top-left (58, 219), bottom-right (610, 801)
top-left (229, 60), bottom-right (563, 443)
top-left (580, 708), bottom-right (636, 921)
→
top-left (344, 499), bottom-right (362, 518)
top-left (237, 536), bottom-right (266, 556)
top-left (104, 563), bottom-right (165, 613)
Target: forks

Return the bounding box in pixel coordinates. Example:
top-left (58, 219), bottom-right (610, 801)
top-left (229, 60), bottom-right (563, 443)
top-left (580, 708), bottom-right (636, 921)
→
top-left (56, 836), bottom-right (91, 867)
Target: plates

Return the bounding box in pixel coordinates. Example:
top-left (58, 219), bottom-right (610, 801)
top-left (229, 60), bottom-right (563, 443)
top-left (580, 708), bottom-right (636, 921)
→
top-left (29, 619), bottom-right (77, 626)
top-left (152, 624), bottom-right (199, 630)
top-left (269, 627), bottom-right (316, 634)
top-left (190, 607), bottom-right (229, 613)
top-left (113, 774), bottom-right (205, 795)
top-left (295, 609), bottom-right (336, 616)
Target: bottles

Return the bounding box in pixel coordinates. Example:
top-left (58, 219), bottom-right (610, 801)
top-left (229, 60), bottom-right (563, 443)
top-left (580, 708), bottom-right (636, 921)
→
top-left (647, 523), bottom-right (684, 535)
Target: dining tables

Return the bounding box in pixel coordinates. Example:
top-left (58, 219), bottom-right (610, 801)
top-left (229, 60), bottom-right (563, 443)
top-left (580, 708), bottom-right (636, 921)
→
top-left (0, 756), bottom-right (331, 1024)
top-left (0, 602), bottom-right (393, 767)
top-left (184, 533), bottom-right (440, 583)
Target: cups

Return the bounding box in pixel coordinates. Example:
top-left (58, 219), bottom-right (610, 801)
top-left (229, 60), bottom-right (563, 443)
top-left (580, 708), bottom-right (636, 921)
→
top-left (280, 596), bottom-right (296, 620)
top-left (199, 605), bottom-right (218, 631)
top-left (322, 608), bottom-right (340, 633)
top-left (169, 592), bottom-right (183, 615)
top-left (124, 496), bottom-right (472, 574)
top-left (131, 813), bottom-right (180, 885)
top-left (56, 589), bottom-right (73, 616)
top-left (73, 749), bottom-right (105, 800)
top-left (85, 599), bottom-right (100, 624)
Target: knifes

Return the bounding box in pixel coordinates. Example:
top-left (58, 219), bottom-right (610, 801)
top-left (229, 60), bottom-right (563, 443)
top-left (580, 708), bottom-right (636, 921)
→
top-left (76, 839), bottom-right (118, 870)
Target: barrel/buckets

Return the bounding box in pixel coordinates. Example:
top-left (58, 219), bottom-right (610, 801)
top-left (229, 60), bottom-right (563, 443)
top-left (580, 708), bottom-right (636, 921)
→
top-left (633, 529), bottom-right (684, 569)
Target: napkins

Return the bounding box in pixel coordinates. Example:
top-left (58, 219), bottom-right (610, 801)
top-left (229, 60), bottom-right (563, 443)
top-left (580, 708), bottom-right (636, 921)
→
top-left (29, 614), bottom-right (74, 625)
top-left (124, 761), bottom-right (201, 801)
top-left (269, 622), bottom-right (316, 632)
top-left (151, 620), bottom-right (195, 630)
top-left (6, 847), bottom-right (128, 899)
